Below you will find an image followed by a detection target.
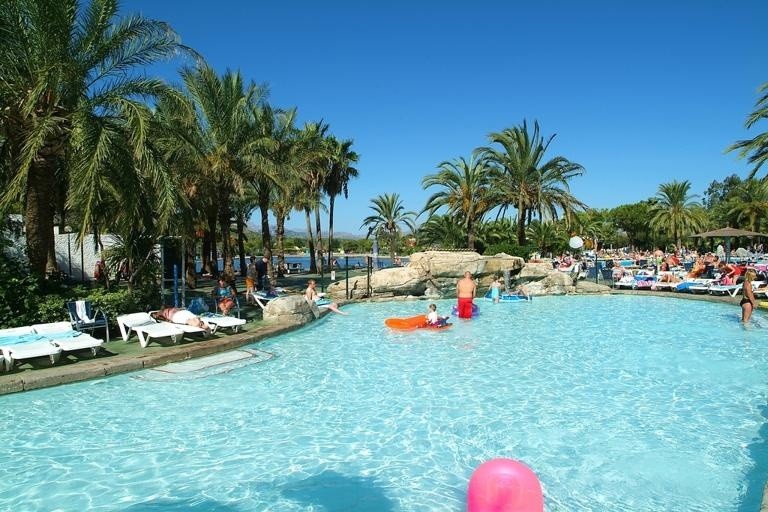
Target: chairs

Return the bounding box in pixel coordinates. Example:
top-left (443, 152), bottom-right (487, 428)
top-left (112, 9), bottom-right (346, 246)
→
top-left (212, 286), bottom-right (243, 319)
top-left (1, 326), bottom-right (58, 373)
top-left (31, 322), bottom-right (106, 365)
top-left (150, 310), bottom-right (208, 341)
top-left (66, 300), bottom-right (109, 343)
top-left (553, 254), bottom-right (768, 299)
top-left (190, 299), bottom-right (245, 335)
top-left (116, 312), bottom-right (184, 348)
top-left (247, 287), bottom-right (327, 312)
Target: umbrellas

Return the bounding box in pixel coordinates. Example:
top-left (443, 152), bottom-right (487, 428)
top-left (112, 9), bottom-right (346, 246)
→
top-left (688, 222), bottom-right (768, 264)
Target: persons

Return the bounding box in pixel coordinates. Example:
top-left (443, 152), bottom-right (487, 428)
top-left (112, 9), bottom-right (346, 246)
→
top-left (552, 241), bottom-right (768, 323)
top-left (427, 304), bottom-right (437, 324)
top-left (395, 257), bottom-right (404, 267)
top-left (150, 255), bottom-right (350, 335)
top-left (456, 271), bottom-right (476, 320)
top-left (488, 272), bottom-right (502, 304)
